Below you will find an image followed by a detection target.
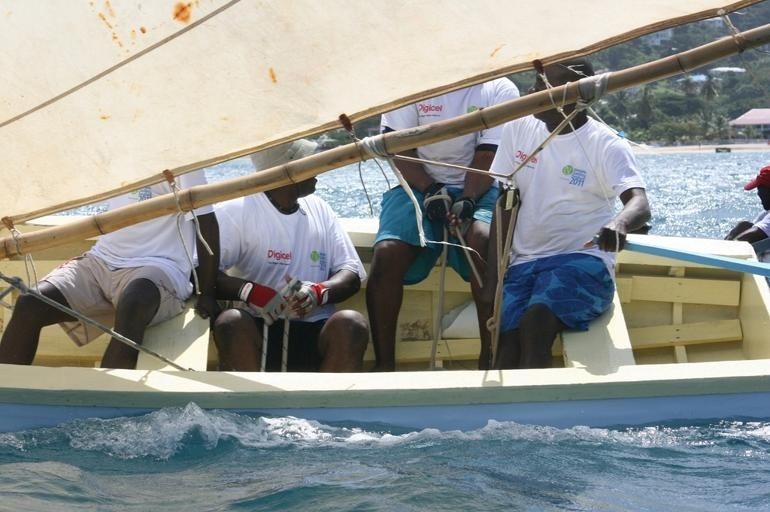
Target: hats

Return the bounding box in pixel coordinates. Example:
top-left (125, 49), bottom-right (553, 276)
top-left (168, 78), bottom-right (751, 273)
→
top-left (250, 139), bottom-right (317, 172)
top-left (745, 165), bottom-right (770, 191)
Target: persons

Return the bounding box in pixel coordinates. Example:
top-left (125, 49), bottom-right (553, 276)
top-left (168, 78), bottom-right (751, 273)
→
top-left (0, 169), bottom-right (223, 370)
top-left (723, 164), bottom-right (770, 289)
top-left (189, 136), bottom-right (371, 372)
top-left (477, 59), bottom-right (651, 368)
top-left (365, 77), bottom-right (522, 372)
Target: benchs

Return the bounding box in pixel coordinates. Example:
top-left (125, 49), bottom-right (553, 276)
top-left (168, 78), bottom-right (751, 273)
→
top-left (134, 294), bottom-right (210, 372)
top-left (559, 282), bottom-right (635, 367)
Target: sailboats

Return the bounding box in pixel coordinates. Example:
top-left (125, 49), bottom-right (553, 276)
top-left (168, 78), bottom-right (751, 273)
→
top-left (0, 2), bottom-right (767, 427)
